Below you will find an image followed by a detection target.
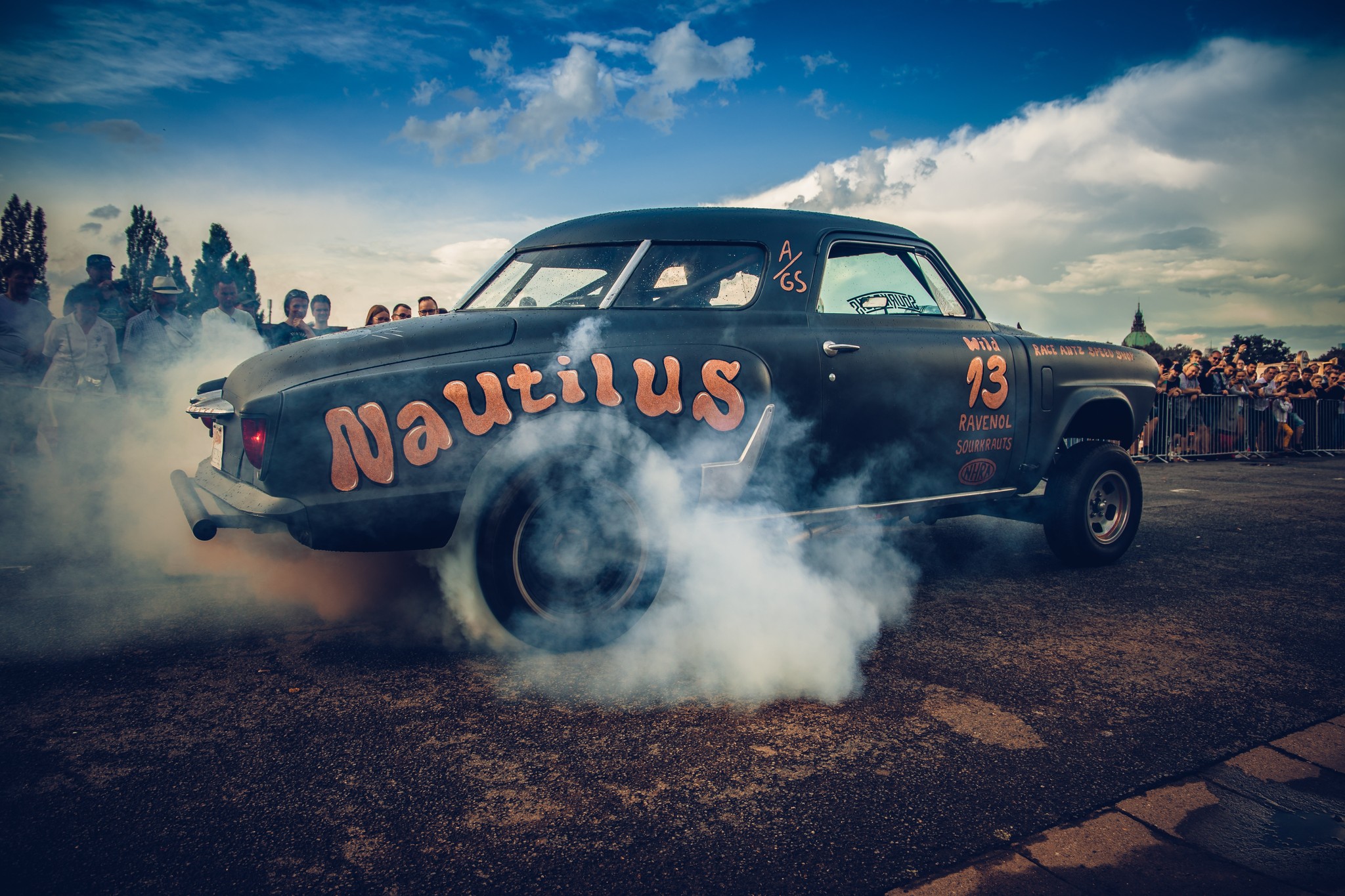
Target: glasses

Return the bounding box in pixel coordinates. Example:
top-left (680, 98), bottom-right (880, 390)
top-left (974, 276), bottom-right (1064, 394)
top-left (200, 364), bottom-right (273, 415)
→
top-left (1330, 376), bottom-right (1338, 381)
top-left (1193, 363), bottom-right (1199, 367)
top-left (289, 290), bottom-right (307, 296)
top-left (419, 309), bottom-right (436, 316)
top-left (394, 313), bottom-right (411, 319)
top-left (372, 316), bottom-right (391, 322)
top-left (1213, 356), bottom-right (1223, 361)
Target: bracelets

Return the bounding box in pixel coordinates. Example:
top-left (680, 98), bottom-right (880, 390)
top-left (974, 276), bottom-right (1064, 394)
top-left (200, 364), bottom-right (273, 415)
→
top-left (1238, 352), bottom-right (1241, 354)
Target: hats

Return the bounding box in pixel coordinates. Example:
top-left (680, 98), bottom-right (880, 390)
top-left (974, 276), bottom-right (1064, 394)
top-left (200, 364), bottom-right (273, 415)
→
top-left (1171, 363), bottom-right (1184, 373)
top-left (1333, 364), bottom-right (1344, 370)
top-left (235, 291), bottom-right (260, 305)
top-left (148, 275), bottom-right (184, 295)
top-left (87, 254), bottom-right (115, 268)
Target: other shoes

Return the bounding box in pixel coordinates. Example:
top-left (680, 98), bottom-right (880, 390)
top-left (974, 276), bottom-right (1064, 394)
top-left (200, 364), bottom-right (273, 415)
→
top-left (1295, 443), bottom-right (1307, 456)
top-left (1132, 447), bottom-right (1291, 463)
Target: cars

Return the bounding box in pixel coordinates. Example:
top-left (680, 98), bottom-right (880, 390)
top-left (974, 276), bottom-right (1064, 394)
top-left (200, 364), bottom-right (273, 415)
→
top-left (166, 204), bottom-right (1163, 658)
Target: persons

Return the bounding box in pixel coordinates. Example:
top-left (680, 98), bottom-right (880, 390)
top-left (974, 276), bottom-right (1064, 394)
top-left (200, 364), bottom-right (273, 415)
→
top-left (309, 294), bottom-right (348, 336)
top-left (265, 289), bottom-right (317, 349)
top-left (0, 261), bottom-right (57, 457)
top-left (364, 305), bottom-right (391, 326)
top-left (200, 277), bottom-right (259, 338)
top-left (1130, 343), bottom-right (1345, 465)
top-left (121, 276), bottom-right (197, 374)
top-left (391, 304), bottom-right (412, 321)
top-left (234, 291), bottom-right (263, 336)
top-left (38, 283), bottom-right (121, 396)
top-left (63, 254), bottom-right (141, 391)
top-left (418, 296), bottom-right (440, 317)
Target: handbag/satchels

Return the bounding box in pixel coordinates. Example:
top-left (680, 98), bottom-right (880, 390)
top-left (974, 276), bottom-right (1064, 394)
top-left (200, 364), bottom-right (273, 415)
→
top-left (64, 325), bottom-right (107, 393)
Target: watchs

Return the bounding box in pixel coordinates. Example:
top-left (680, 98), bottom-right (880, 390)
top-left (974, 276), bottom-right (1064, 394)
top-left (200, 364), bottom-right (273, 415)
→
top-left (1197, 389), bottom-right (1200, 394)
top-left (1299, 394), bottom-right (1301, 398)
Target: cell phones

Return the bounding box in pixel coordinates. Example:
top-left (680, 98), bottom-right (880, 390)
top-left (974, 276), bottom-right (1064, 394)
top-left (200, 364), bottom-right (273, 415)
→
top-left (1259, 382), bottom-right (1268, 387)
top-left (1337, 381), bottom-right (1344, 385)
top-left (1233, 379), bottom-right (1243, 384)
top-left (1212, 368), bottom-right (1224, 372)
top-left (1226, 349), bottom-right (1234, 354)
top-left (1286, 382), bottom-right (1292, 385)
top-left (1163, 370), bottom-right (1168, 376)
